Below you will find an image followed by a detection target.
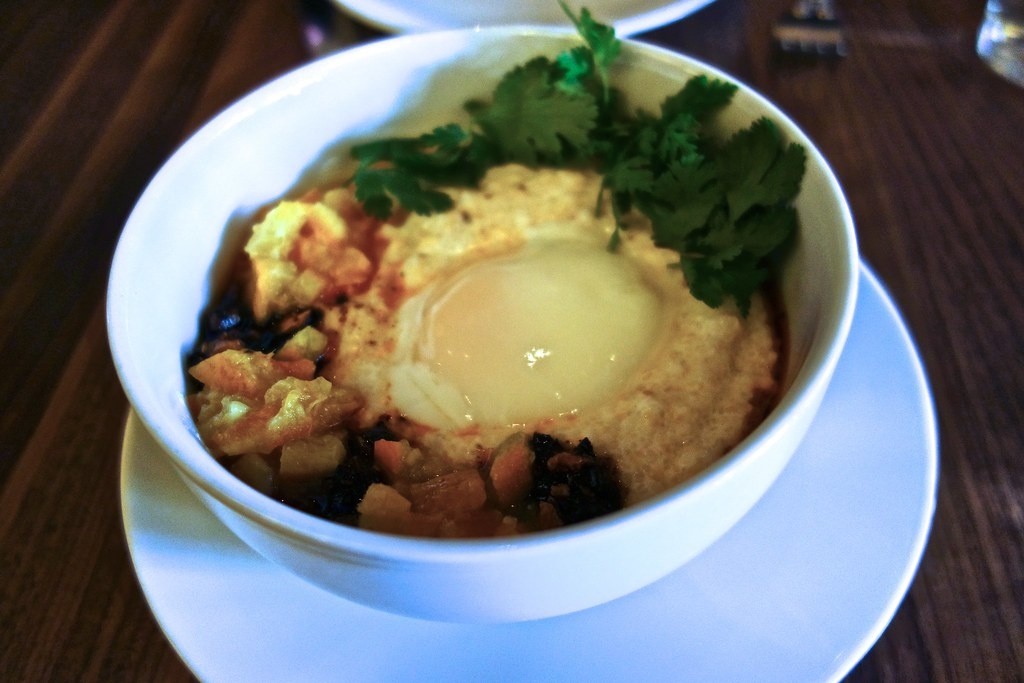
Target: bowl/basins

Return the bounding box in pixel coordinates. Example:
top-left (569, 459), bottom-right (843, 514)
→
top-left (105, 21), bottom-right (863, 624)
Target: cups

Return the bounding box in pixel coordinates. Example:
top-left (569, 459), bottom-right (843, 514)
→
top-left (976, 0), bottom-right (1024, 83)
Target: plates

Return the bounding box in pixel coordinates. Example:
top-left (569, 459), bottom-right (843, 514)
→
top-left (119, 263), bottom-right (940, 683)
top-left (335, 0), bottom-right (713, 38)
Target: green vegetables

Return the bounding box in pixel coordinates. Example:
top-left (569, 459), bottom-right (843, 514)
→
top-left (349, 0), bottom-right (806, 322)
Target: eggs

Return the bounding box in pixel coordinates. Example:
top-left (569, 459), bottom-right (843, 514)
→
top-left (317, 164), bottom-right (775, 500)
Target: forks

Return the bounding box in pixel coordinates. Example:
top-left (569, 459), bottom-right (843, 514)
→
top-left (768, 0), bottom-right (850, 57)
